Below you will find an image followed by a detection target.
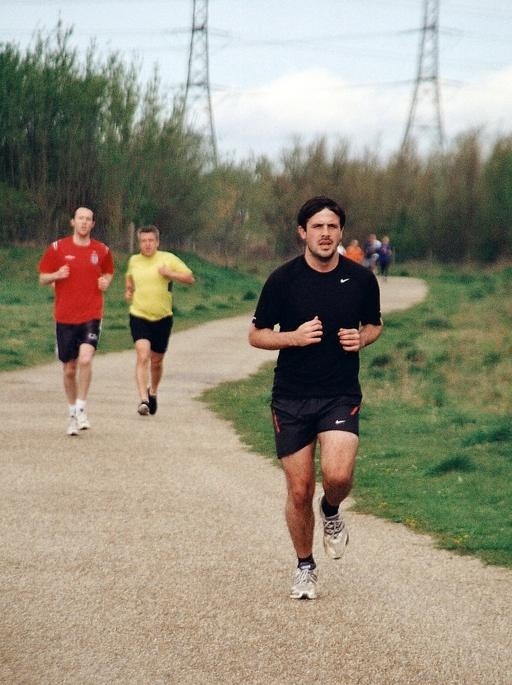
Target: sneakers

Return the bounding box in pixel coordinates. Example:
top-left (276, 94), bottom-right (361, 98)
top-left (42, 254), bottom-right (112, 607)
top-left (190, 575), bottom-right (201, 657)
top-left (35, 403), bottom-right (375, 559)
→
top-left (138, 388), bottom-right (156, 415)
top-left (318, 495), bottom-right (348, 559)
top-left (67, 416), bottom-right (89, 435)
top-left (290, 563), bottom-right (318, 599)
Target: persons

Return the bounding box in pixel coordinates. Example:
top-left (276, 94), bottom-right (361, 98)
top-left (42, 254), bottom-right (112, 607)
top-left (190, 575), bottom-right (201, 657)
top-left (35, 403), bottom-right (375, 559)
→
top-left (337, 234), bottom-right (392, 276)
top-left (248, 195), bottom-right (385, 599)
top-left (125, 225), bottom-right (192, 416)
top-left (36, 205), bottom-right (115, 436)
